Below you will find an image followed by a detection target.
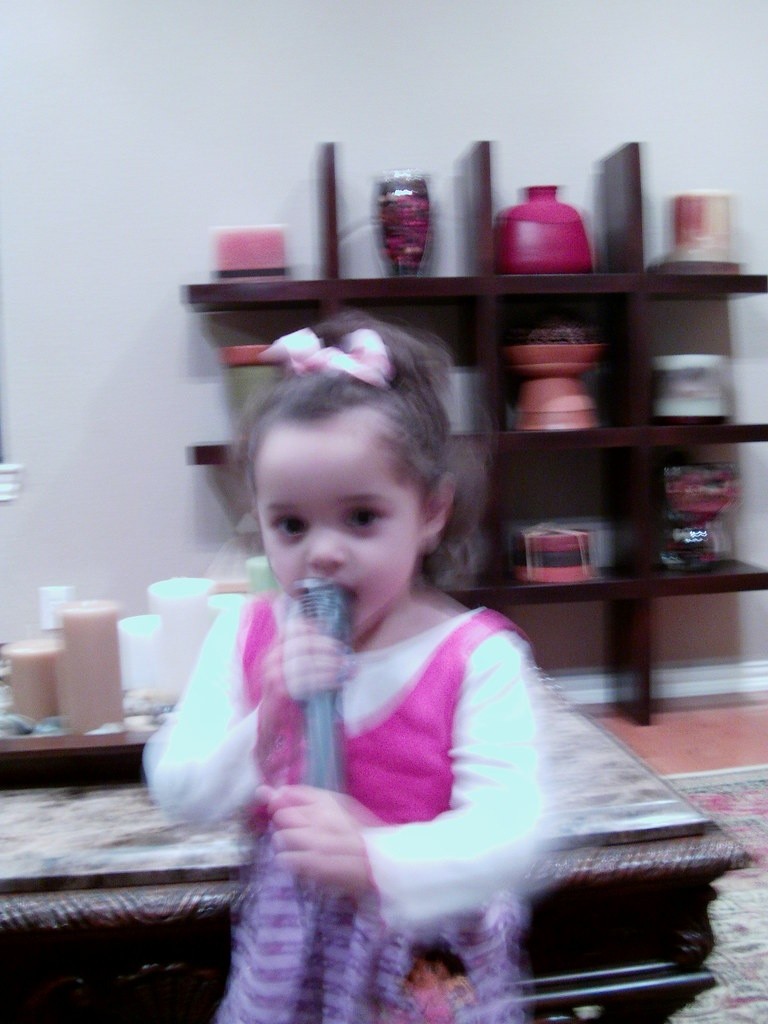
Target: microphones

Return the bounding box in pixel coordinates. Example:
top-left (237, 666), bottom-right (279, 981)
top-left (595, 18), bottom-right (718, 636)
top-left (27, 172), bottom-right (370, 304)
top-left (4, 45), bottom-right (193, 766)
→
top-left (291, 577), bottom-right (352, 791)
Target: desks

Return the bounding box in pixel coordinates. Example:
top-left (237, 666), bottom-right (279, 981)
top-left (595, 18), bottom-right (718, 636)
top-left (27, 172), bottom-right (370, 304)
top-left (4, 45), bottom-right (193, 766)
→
top-left (0, 659), bottom-right (754, 1024)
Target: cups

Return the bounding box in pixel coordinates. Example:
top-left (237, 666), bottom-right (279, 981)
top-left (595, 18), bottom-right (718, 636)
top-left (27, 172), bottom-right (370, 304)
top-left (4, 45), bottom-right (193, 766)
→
top-left (147, 578), bottom-right (217, 702)
top-left (0, 638), bottom-right (59, 720)
top-left (117, 614), bottom-right (162, 711)
top-left (59, 599), bottom-right (126, 737)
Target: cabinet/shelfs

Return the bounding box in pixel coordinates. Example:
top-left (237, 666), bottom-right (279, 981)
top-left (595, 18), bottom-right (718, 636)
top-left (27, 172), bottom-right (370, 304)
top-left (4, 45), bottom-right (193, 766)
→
top-left (186, 141), bottom-right (768, 729)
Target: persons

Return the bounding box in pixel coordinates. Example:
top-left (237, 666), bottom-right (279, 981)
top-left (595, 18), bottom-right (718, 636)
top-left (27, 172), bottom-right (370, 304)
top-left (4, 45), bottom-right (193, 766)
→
top-left (142, 315), bottom-right (544, 1024)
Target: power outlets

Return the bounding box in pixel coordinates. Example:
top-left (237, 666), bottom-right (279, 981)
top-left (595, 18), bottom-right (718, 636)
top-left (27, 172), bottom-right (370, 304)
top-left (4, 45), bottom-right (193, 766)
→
top-left (38, 586), bottom-right (65, 630)
